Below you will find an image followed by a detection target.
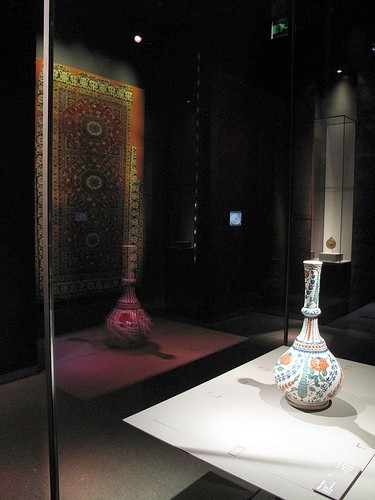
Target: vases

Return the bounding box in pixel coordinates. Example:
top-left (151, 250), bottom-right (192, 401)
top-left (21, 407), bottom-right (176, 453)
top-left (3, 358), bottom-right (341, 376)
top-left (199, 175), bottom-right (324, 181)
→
top-left (102, 242), bottom-right (154, 351)
top-left (274, 259), bottom-right (346, 414)
